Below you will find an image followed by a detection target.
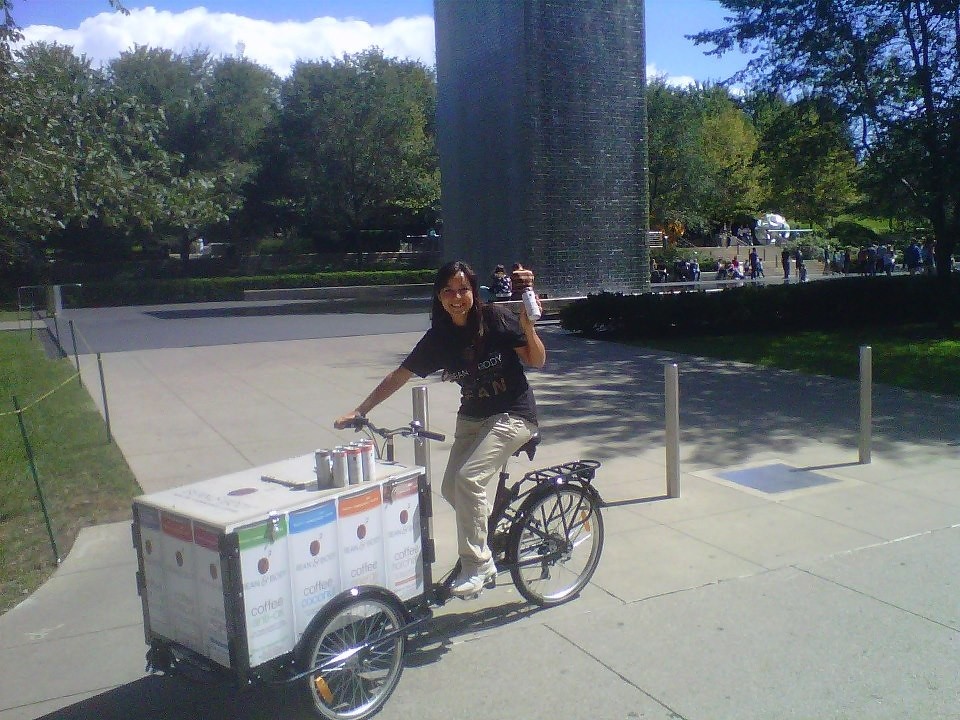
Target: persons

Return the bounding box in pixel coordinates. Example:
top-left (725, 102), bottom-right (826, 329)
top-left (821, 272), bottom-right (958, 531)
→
top-left (717, 247), bottom-right (766, 279)
top-left (510, 262), bottom-right (534, 300)
top-left (719, 224), bottom-right (753, 247)
top-left (795, 249), bottom-right (803, 269)
top-left (487, 265), bottom-right (512, 302)
top-left (822, 237), bottom-right (935, 276)
top-left (650, 259), bottom-right (701, 283)
top-left (781, 245), bottom-right (790, 278)
top-left (333, 261), bottom-right (546, 596)
top-left (799, 263), bottom-right (807, 282)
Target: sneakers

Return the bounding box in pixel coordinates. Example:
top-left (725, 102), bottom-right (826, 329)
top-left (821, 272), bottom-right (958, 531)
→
top-left (450, 557), bottom-right (497, 596)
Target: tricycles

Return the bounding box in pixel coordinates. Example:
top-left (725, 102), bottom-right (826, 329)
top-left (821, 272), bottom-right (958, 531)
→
top-left (132, 415), bottom-right (609, 720)
